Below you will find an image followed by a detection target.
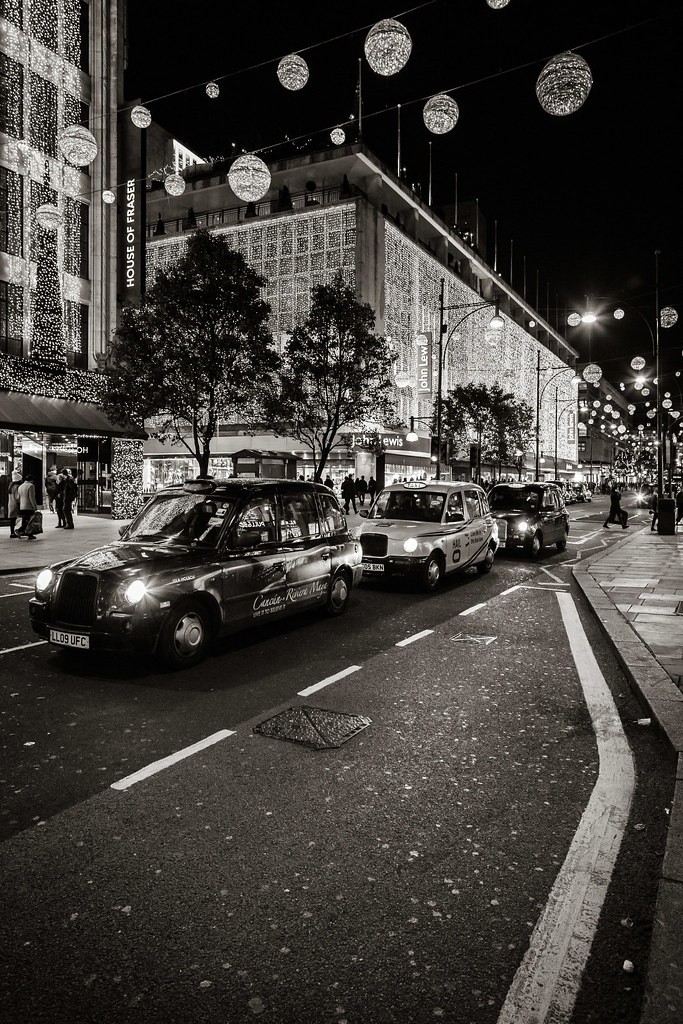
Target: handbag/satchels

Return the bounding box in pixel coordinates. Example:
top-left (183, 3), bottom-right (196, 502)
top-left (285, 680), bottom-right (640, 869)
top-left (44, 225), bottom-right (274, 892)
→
top-left (25, 512), bottom-right (43, 535)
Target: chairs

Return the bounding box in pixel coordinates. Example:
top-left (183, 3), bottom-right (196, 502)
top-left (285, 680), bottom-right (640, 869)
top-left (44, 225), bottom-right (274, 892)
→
top-left (238, 531), bottom-right (261, 547)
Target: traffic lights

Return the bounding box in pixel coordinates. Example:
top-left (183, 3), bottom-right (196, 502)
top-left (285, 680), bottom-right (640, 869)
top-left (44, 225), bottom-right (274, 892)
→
top-left (431, 436), bottom-right (439, 464)
top-left (445, 438), bottom-right (456, 465)
top-left (469, 444), bottom-right (478, 468)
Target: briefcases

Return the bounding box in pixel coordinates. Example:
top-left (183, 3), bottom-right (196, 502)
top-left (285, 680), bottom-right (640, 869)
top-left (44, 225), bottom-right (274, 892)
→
top-left (609, 510), bottom-right (628, 524)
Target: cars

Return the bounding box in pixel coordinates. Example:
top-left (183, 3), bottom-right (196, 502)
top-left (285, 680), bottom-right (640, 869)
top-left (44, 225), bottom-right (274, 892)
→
top-left (477, 482), bottom-right (570, 559)
top-left (636, 483), bottom-right (660, 509)
top-left (663, 483), bottom-right (679, 498)
top-left (29, 477), bottom-right (363, 668)
top-left (544, 479), bottom-right (593, 505)
top-left (350, 480), bottom-right (500, 592)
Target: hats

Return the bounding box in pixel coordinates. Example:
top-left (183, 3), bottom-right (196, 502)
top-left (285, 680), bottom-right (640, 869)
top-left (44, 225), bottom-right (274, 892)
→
top-left (12, 471), bottom-right (23, 482)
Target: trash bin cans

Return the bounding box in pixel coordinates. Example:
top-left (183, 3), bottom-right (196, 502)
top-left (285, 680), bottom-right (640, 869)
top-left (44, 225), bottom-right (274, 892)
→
top-left (657, 498), bottom-right (677, 535)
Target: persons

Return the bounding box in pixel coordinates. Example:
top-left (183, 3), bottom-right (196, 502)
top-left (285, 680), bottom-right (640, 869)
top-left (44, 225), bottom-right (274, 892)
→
top-left (591, 480), bottom-right (683, 531)
top-left (297, 472), bottom-right (440, 519)
top-left (8, 466), bottom-right (77, 539)
top-left (455, 475), bottom-right (514, 492)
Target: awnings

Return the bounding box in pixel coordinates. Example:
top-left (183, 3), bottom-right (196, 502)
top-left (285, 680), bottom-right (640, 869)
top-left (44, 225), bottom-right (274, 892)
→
top-left (0, 389), bottom-right (149, 440)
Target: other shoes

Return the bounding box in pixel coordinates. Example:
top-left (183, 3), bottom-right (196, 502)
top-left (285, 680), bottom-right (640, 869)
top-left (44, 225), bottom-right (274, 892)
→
top-left (603, 525), bottom-right (610, 530)
top-left (56, 526), bottom-right (64, 528)
top-left (623, 526), bottom-right (629, 529)
top-left (14, 530), bottom-right (22, 539)
top-left (64, 526), bottom-right (74, 529)
top-left (28, 536), bottom-right (36, 539)
top-left (10, 535), bottom-right (17, 538)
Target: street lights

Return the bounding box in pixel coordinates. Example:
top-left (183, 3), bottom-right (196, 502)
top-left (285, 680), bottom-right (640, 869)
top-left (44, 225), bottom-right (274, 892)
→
top-left (513, 368), bottom-right (581, 482)
top-left (406, 300), bottom-right (505, 480)
top-left (539, 399), bottom-right (601, 480)
top-left (582, 294), bottom-right (663, 498)
top-left (479, 420), bottom-right (485, 485)
